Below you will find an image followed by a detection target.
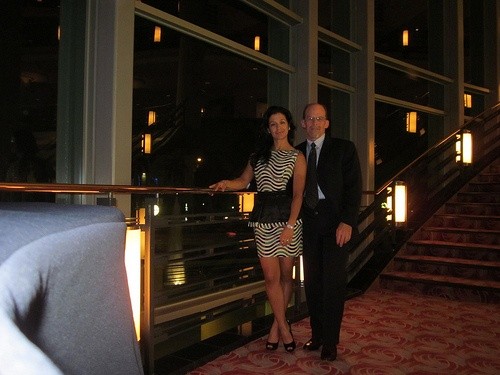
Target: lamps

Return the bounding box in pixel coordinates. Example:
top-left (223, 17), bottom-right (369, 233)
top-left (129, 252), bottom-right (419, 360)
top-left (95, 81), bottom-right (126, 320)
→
top-left (455, 130), bottom-right (475, 165)
top-left (238, 194), bottom-right (257, 217)
top-left (141, 134), bottom-right (152, 155)
top-left (406, 111), bottom-right (418, 133)
top-left (124, 216), bottom-right (142, 341)
top-left (394, 179), bottom-right (410, 223)
top-left (464, 93), bottom-right (472, 108)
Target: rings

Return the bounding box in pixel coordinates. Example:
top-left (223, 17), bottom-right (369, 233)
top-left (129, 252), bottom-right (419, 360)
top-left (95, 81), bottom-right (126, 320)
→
top-left (287, 240), bottom-right (288, 241)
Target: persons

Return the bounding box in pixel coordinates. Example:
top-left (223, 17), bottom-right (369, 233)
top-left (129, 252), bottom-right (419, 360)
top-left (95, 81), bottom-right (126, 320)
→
top-left (209, 105), bottom-right (307, 352)
top-left (293, 104), bottom-right (362, 360)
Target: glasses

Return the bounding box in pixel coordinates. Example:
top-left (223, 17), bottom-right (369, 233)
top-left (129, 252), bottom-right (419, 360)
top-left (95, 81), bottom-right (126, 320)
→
top-left (304, 116), bottom-right (326, 122)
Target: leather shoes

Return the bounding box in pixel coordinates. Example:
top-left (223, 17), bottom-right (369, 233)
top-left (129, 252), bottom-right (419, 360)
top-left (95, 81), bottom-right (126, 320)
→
top-left (303, 338), bottom-right (321, 350)
top-left (320, 343), bottom-right (337, 361)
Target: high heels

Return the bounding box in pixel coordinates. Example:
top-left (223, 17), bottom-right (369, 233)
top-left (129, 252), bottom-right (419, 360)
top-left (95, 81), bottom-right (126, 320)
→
top-left (283, 321), bottom-right (296, 352)
top-left (266, 336), bottom-right (279, 349)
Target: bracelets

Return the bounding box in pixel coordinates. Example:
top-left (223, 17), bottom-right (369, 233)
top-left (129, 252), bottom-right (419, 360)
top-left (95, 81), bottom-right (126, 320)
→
top-left (286, 224), bottom-right (294, 229)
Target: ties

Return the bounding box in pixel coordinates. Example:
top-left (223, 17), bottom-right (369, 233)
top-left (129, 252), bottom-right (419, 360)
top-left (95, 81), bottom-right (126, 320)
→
top-left (305, 142), bottom-right (319, 209)
